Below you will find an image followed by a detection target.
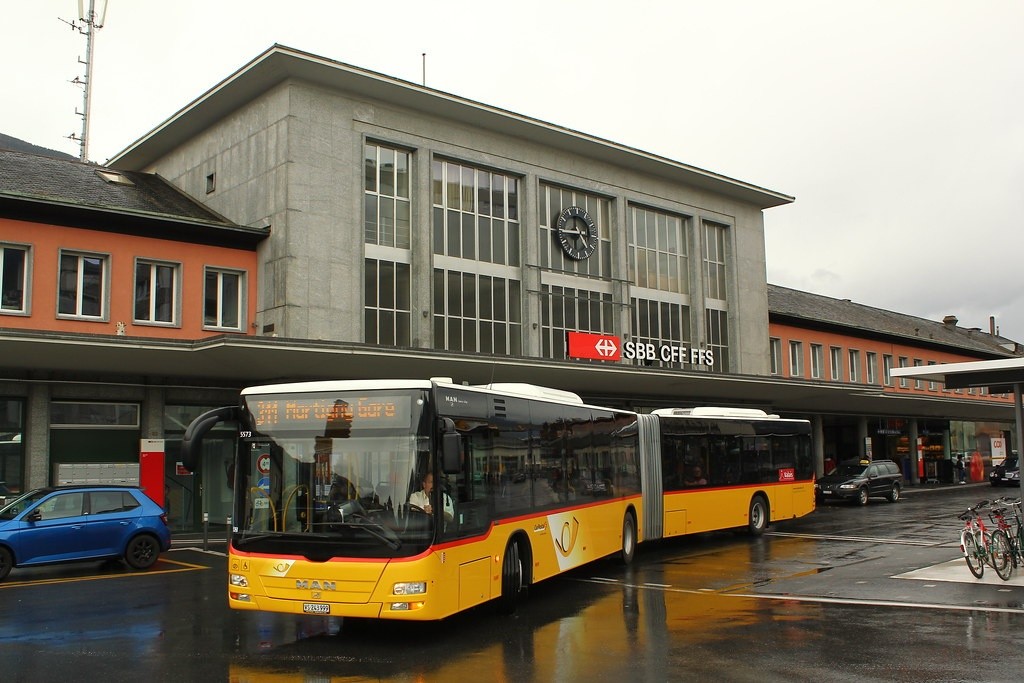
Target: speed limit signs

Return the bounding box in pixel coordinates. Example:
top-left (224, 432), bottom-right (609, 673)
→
top-left (256, 453), bottom-right (270, 474)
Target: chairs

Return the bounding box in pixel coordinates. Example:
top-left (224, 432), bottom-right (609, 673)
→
top-left (67, 496), bottom-right (82, 517)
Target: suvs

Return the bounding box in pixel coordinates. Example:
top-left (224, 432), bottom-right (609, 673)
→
top-left (0, 483), bottom-right (173, 582)
top-left (814, 458), bottom-right (903, 507)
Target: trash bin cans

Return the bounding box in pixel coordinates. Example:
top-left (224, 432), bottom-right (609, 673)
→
top-left (926, 458), bottom-right (940, 484)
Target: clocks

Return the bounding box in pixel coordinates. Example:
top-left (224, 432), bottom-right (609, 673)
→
top-left (557, 206), bottom-right (597, 259)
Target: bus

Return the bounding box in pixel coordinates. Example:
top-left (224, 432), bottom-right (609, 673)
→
top-left (179, 375), bottom-right (815, 630)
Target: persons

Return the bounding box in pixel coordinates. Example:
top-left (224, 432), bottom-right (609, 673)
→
top-left (326, 463), bottom-right (375, 508)
top-left (409, 472), bottom-right (454, 533)
top-left (956, 454), bottom-right (968, 484)
top-left (480, 471), bottom-right (507, 498)
top-left (683, 466), bottom-right (707, 486)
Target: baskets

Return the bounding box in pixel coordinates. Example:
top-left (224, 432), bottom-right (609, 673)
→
top-left (988, 510), bottom-right (1016, 524)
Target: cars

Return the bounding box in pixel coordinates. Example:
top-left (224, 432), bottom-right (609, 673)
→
top-left (989, 453), bottom-right (1020, 488)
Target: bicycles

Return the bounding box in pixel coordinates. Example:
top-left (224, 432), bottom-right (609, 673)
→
top-left (958, 496), bottom-right (1024, 581)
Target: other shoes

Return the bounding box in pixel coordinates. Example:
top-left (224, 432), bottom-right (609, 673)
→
top-left (958, 481), bottom-right (966, 485)
